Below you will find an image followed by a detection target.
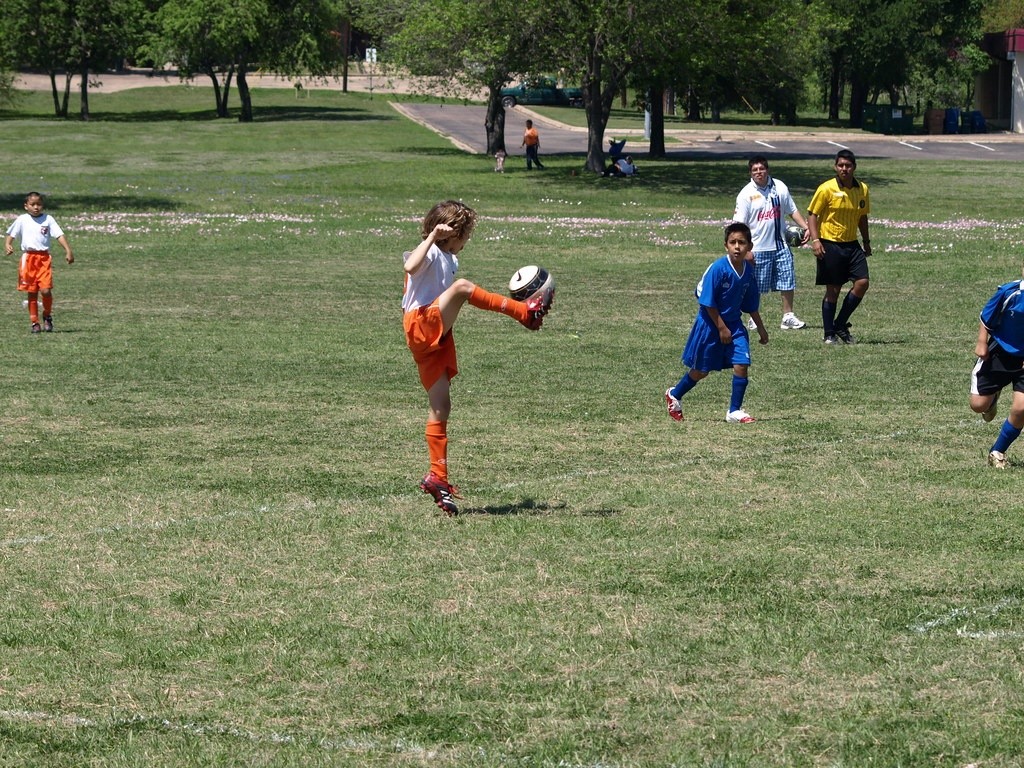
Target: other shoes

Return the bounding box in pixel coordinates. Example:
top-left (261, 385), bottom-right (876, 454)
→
top-left (609, 173), bottom-right (614, 177)
top-left (600, 172), bottom-right (604, 176)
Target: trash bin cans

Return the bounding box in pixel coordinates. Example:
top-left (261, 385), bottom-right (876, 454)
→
top-left (861, 104), bottom-right (914, 135)
top-left (927, 109), bottom-right (986, 135)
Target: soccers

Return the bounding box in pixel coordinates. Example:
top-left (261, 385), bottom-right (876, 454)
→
top-left (784, 225), bottom-right (804, 247)
top-left (507, 264), bottom-right (557, 309)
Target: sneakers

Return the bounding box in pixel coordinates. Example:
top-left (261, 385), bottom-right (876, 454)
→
top-left (419, 473), bottom-right (463, 516)
top-left (31, 323), bottom-right (41, 334)
top-left (43, 316), bottom-right (53, 331)
top-left (726, 409), bottom-right (756, 423)
top-left (781, 317), bottom-right (806, 329)
top-left (665, 387), bottom-right (683, 421)
top-left (833, 320), bottom-right (854, 344)
top-left (824, 335), bottom-right (841, 345)
top-left (520, 287), bottom-right (554, 330)
top-left (987, 449), bottom-right (1011, 470)
top-left (982, 389), bottom-right (1000, 422)
top-left (748, 319), bottom-right (758, 331)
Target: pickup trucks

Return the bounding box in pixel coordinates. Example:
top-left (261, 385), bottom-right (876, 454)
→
top-left (501, 75), bottom-right (585, 109)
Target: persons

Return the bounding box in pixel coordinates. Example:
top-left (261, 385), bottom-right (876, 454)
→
top-left (732, 158), bottom-right (810, 333)
top-left (808, 149), bottom-right (872, 347)
top-left (494, 150), bottom-right (507, 174)
top-left (970, 260), bottom-right (1024, 469)
top-left (517, 118), bottom-right (544, 171)
top-left (600, 156), bottom-right (637, 178)
top-left (6, 191), bottom-right (74, 332)
top-left (401, 200), bottom-right (555, 515)
top-left (665, 222), bottom-right (768, 426)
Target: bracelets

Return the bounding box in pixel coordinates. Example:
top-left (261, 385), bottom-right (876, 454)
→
top-left (812, 239), bottom-right (819, 244)
top-left (804, 228), bottom-right (809, 231)
